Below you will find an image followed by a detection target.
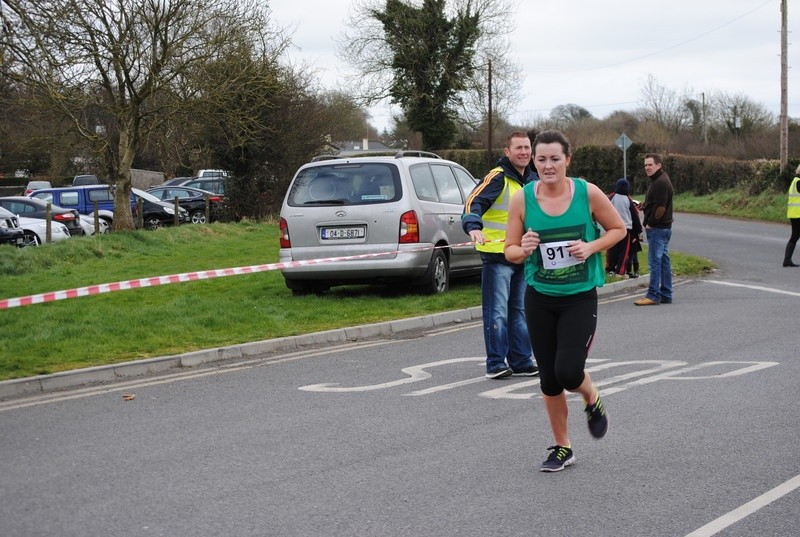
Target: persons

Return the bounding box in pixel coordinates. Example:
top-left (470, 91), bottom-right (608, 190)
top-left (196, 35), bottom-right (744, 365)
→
top-left (605, 178), bottom-right (644, 278)
top-left (783, 163), bottom-right (800, 267)
top-left (461, 132), bottom-right (539, 379)
top-left (634, 153), bottom-right (673, 305)
top-left (504, 128), bottom-right (627, 472)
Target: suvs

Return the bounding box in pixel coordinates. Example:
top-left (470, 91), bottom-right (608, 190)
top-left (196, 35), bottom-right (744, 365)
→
top-left (73, 174), bottom-right (98, 186)
top-left (27, 185), bottom-right (138, 234)
top-left (155, 170), bottom-right (234, 196)
top-left (278, 150), bottom-right (483, 295)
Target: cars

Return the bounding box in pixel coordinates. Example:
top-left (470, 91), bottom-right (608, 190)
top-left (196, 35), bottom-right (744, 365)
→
top-left (0, 196), bottom-right (81, 236)
top-left (0, 206), bottom-right (24, 244)
top-left (132, 189), bottom-right (189, 232)
top-left (17, 217), bottom-right (70, 249)
top-left (79, 214), bottom-right (109, 237)
top-left (23, 181), bottom-right (51, 197)
top-left (144, 186), bottom-right (225, 225)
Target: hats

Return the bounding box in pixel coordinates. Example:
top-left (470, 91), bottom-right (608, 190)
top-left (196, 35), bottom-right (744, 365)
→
top-left (615, 178), bottom-right (629, 193)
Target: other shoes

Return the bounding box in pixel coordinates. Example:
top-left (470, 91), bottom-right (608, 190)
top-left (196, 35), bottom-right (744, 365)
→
top-left (783, 260), bottom-right (799, 267)
top-left (607, 271), bottom-right (638, 278)
top-left (634, 298), bottom-right (660, 304)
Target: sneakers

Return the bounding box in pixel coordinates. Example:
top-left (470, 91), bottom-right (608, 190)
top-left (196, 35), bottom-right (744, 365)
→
top-left (485, 367), bottom-right (513, 378)
top-left (513, 365), bottom-right (539, 376)
top-left (583, 384), bottom-right (607, 438)
top-left (539, 445), bottom-right (575, 471)
